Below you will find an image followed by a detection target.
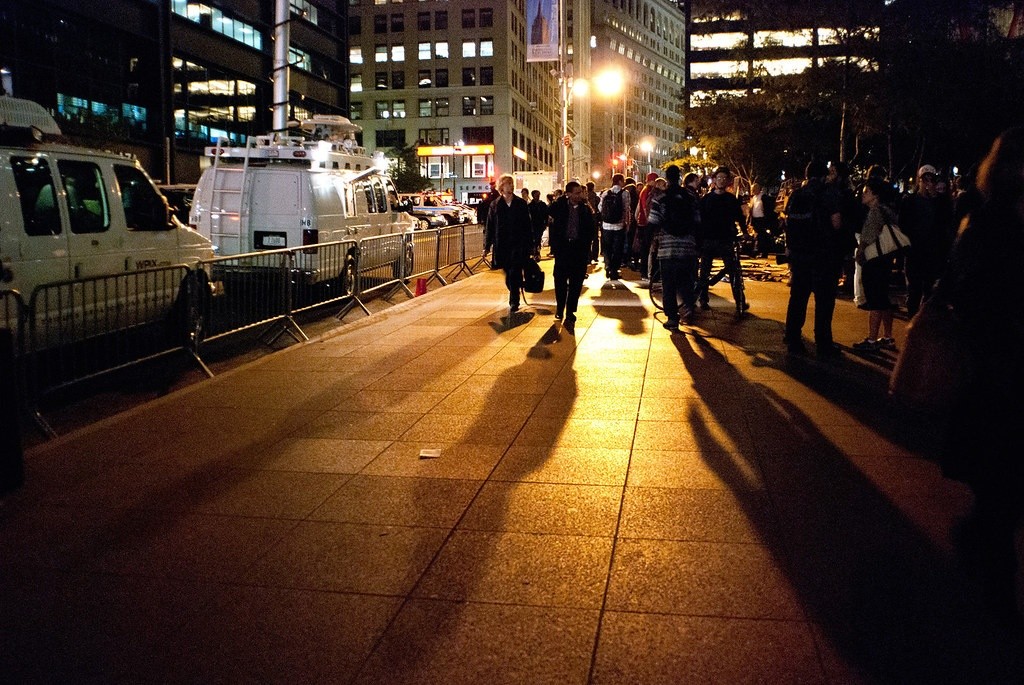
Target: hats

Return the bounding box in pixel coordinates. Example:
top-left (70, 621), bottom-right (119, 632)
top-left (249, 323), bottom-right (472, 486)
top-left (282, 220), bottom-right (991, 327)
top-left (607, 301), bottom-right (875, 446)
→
top-left (648, 173), bottom-right (658, 180)
top-left (919, 164), bottom-right (936, 177)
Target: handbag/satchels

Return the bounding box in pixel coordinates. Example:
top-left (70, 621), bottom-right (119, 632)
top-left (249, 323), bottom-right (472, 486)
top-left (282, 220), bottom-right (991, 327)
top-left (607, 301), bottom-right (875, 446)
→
top-left (891, 296), bottom-right (967, 408)
top-left (853, 224), bottom-right (910, 310)
top-left (524, 260), bottom-right (544, 293)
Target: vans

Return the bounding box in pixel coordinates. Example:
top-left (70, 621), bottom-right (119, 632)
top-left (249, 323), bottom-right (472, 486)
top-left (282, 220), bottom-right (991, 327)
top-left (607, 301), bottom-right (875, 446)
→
top-left (0, 95), bottom-right (214, 383)
top-left (187, 114), bottom-right (421, 300)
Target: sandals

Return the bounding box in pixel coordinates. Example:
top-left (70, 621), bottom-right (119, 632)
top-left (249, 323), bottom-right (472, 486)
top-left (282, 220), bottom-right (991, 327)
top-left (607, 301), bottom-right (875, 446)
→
top-left (849, 338), bottom-right (879, 352)
top-left (879, 337), bottom-right (896, 349)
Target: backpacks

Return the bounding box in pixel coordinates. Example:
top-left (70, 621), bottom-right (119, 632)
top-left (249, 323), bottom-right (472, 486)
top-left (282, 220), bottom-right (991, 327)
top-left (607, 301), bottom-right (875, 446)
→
top-left (664, 188), bottom-right (695, 238)
top-left (601, 190), bottom-right (624, 223)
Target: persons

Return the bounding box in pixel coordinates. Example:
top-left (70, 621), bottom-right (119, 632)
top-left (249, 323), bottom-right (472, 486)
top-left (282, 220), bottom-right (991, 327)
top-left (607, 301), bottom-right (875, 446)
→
top-left (740, 171), bottom-right (793, 263)
top-left (847, 164), bottom-right (974, 351)
top-left (480, 176), bottom-right (600, 322)
top-left (936, 126), bottom-right (1023, 637)
top-left (598, 165), bottom-right (748, 329)
top-left (784, 161), bottom-right (851, 357)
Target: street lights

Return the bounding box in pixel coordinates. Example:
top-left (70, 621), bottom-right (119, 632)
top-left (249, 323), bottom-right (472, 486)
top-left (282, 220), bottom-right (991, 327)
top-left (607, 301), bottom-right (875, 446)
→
top-left (644, 141), bottom-right (654, 174)
top-left (597, 65), bottom-right (622, 179)
top-left (560, 77), bottom-right (589, 191)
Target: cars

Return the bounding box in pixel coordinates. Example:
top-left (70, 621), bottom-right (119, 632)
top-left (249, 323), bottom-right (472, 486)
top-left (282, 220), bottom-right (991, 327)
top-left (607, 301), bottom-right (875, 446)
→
top-left (395, 192), bottom-right (479, 231)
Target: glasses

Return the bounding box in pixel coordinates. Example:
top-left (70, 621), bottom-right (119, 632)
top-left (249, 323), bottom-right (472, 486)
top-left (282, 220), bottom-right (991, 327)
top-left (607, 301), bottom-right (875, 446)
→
top-left (717, 177), bottom-right (726, 181)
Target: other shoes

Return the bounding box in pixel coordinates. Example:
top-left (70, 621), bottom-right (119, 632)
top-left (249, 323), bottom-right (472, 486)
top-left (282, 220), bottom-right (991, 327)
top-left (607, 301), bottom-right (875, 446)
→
top-left (555, 308), bottom-right (563, 318)
top-left (606, 268), bottom-right (614, 278)
top-left (701, 300), bottom-right (710, 310)
top-left (683, 311), bottom-right (693, 319)
top-left (817, 342), bottom-right (840, 354)
top-left (787, 345), bottom-right (811, 358)
top-left (642, 274), bottom-right (648, 281)
top-left (736, 302), bottom-right (750, 311)
top-left (566, 312), bottom-right (576, 321)
top-left (663, 318), bottom-right (679, 330)
top-left (511, 303), bottom-right (518, 311)
